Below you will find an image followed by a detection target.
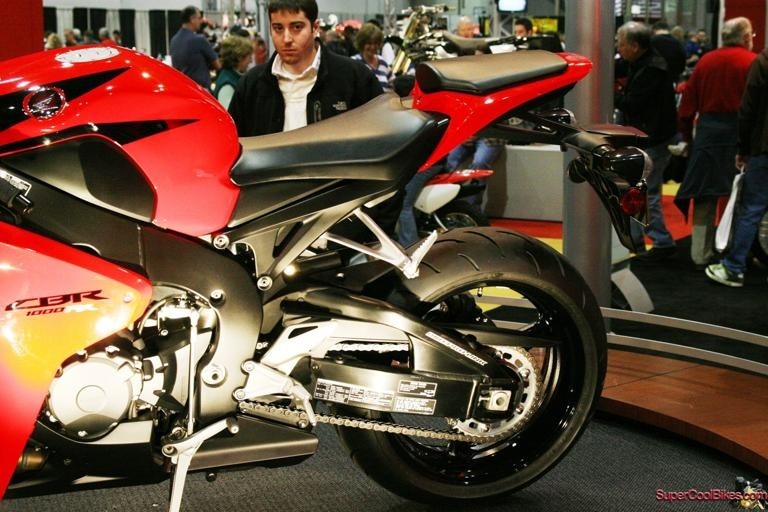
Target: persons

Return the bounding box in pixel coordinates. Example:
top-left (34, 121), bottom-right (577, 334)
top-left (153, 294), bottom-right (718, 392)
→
top-left (677, 18), bottom-right (758, 264)
top-left (228, 0), bottom-right (384, 137)
top-left (613, 21), bottom-right (679, 261)
top-left (515, 18), bottom-right (533, 36)
top-left (613, 20), bottom-right (711, 184)
top-left (317, 14), bottom-right (396, 94)
top-left (442, 15), bottom-right (493, 57)
top-left (705, 49), bottom-right (768, 288)
top-left (44, 28), bottom-right (121, 52)
top-left (170, 5), bottom-right (268, 111)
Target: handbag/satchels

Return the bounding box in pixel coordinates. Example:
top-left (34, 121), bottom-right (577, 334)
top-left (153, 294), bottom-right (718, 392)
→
top-left (715, 166), bottom-right (746, 254)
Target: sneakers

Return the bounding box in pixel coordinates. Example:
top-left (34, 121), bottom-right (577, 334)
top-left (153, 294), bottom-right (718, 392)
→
top-left (705, 263), bottom-right (744, 287)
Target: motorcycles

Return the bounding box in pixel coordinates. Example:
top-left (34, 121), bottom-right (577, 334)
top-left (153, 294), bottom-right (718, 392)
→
top-left (0, 43), bottom-right (653, 511)
top-left (379, 0), bottom-right (565, 110)
